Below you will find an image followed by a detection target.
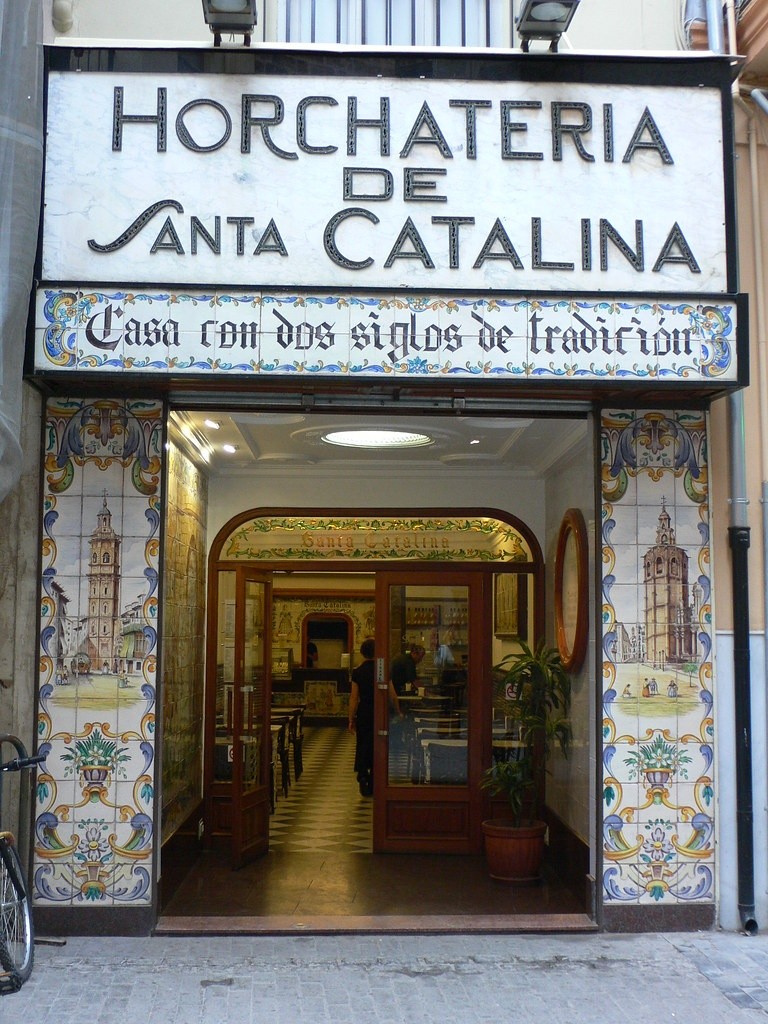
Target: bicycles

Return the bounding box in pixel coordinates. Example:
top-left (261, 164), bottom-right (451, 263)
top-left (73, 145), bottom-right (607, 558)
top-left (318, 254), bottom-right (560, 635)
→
top-left (0, 735), bottom-right (47, 983)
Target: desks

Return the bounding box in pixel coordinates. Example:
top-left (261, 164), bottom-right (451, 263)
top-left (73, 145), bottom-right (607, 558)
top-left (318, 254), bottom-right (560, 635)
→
top-left (270, 707), bottom-right (305, 781)
top-left (413, 718), bottom-right (501, 785)
top-left (414, 728), bottom-right (512, 784)
top-left (420, 738), bottom-right (534, 785)
top-left (216, 714), bottom-right (294, 800)
top-left (408, 709), bottom-right (468, 778)
top-left (216, 724), bottom-right (282, 803)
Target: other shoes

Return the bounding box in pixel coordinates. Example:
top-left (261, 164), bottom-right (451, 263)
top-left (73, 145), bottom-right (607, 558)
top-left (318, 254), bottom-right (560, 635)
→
top-left (360, 778), bottom-right (367, 795)
top-left (368, 784), bottom-right (373, 795)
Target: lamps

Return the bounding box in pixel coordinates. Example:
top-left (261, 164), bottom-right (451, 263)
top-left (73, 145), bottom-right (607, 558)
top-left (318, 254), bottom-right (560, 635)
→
top-left (514, 0), bottom-right (581, 53)
top-left (202, 0), bottom-right (258, 47)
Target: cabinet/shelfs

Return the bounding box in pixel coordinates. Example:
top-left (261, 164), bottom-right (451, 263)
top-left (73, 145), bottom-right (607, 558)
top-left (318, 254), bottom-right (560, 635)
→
top-left (406, 605), bottom-right (468, 652)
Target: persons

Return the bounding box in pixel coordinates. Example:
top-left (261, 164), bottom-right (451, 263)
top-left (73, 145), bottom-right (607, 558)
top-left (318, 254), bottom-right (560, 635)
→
top-left (390, 645), bottom-right (425, 711)
top-left (347, 638), bottom-right (405, 799)
top-left (306, 632), bottom-right (318, 667)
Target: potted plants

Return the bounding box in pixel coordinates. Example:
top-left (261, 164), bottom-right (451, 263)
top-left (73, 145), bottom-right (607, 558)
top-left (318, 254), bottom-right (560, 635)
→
top-left (476, 634), bottom-right (574, 877)
